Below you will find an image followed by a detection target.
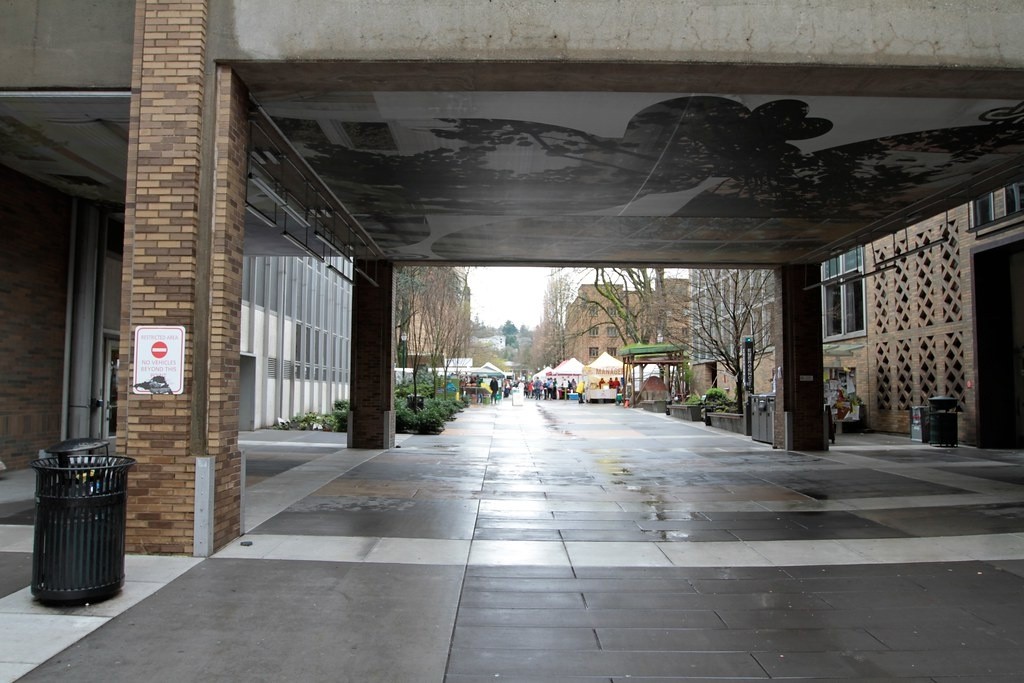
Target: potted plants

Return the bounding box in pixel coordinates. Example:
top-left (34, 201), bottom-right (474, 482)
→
top-left (836, 401), bottom-right (849, 420)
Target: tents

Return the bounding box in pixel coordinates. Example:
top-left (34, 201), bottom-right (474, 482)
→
top-left (553, 357), bottom-right (586, 390)
top-left (582, 352), bottom-right (633, 404)
top-left (546, 358), bottom-right (579, 398)
top-left (533, 366), bottom-right (552, 381)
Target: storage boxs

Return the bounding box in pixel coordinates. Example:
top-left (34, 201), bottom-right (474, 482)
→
top-left (566, 393), bottom-right (579, 400)
top-left (601, 383), bottom-right (609, 389)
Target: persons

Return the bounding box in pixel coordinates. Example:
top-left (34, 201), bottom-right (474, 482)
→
top-left (576, 381), bottom-right (585, 403)
top-left (470, 373), bottom-right (484, 403)
top-left (503, 379), bottom-right (534, 399)
top-left (599, 377), bottom-right (620, 389)
top-left (562, 378), bottom-right (576, 392)
top-left (534, 376), bottom-right (558, 400)
top-left (490, 376), bottom-right (498, 404)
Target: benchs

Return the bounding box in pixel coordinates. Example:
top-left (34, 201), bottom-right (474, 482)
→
top-left (706, 412), bottom-right (744, 434)
top-left (585, 389), bottom-right (617, 404)
top-left (640, 400), bottom-right (666, 413)
top-left (667, 403), bottom-right (698, 421)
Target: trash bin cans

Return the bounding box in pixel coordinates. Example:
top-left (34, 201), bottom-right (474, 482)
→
top-left (28, 439), bottom-right (137, 606)
top-left (928, 396), bottom-right (959, 448)
top-left (666, 400), bottom-right (673, 415)
top-left (705, 406), bottom-right (717, 426)
top-left (407, 395), bottom-right (426, 414)
